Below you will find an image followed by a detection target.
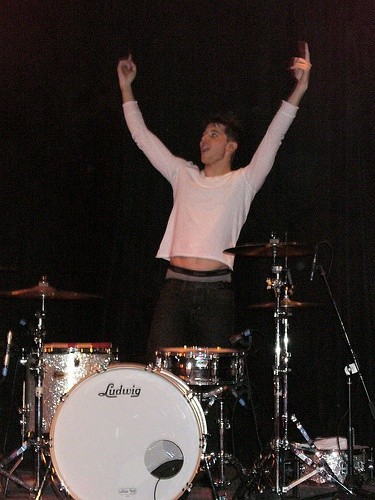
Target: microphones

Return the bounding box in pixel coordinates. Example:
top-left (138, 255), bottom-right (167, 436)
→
top-left (310, 243), bottom-right (318, 281)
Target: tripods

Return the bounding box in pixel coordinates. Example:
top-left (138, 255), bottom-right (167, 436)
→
top-left (1, 296), bottom-right (75, 500)
top-left (194, 252), bottom-right (356, 499)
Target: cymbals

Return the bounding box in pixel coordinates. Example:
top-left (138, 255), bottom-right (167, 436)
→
top-left (0, 275), bottom-right (104, 301)
top-left (244, 286), bottom-right (331, 310)
top-left (222, 231), bottom-right (332, 259)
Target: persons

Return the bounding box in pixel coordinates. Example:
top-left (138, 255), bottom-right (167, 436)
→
top-left (117, 42), bottom-right (312, 486)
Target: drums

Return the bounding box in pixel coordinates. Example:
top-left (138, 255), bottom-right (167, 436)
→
top-left (270, 439), bottom-right (372, 488)
top-left (20, 342), bottom-right (119, 444)
top-left (49, 363), bottom-right (208, 500)
top-left (155, 346), bottom-right (246, 386)
